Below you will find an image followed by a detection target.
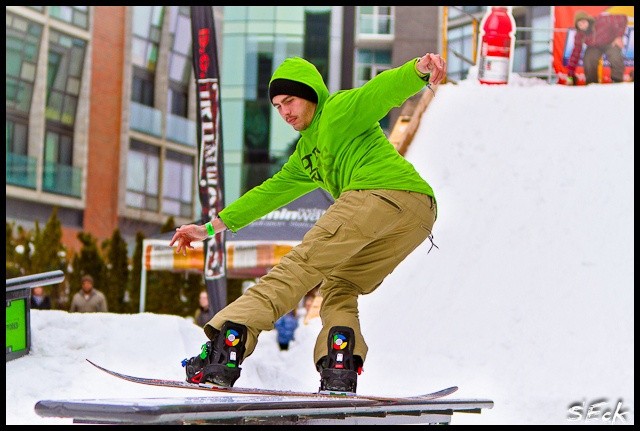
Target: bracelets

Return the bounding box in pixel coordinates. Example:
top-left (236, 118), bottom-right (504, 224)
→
top-left (204, 221), bottom-right (215, 237)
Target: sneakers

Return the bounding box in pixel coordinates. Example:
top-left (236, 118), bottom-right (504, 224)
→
top-left (181, 321), bottom-right (247, 389)
top-left (314, 328), bottom-right (363, 392)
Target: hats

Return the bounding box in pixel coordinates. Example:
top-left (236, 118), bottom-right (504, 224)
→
top-left (269, 79), bottom-right (318, 103)
top-left (82, 274), bottom-right (93, 283)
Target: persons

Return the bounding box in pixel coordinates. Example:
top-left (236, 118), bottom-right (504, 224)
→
top-left (304, 294), bottom-right (315, 311)
top-left (31, 285), bottom-right (51, 309)
top-left (70, 274), bottom-right (108, 312)
top-left (169, 52), bottom-right (448, 391)
top-left (194, 290), bottom-right (211, 327)
top-left (274, 310), bottom-right (300, 351)
top-left (564, 10), bottom-right (628, 84)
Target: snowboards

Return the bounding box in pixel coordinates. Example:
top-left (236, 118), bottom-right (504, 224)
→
top-left (85, 359), bottom-right (458, 402)
